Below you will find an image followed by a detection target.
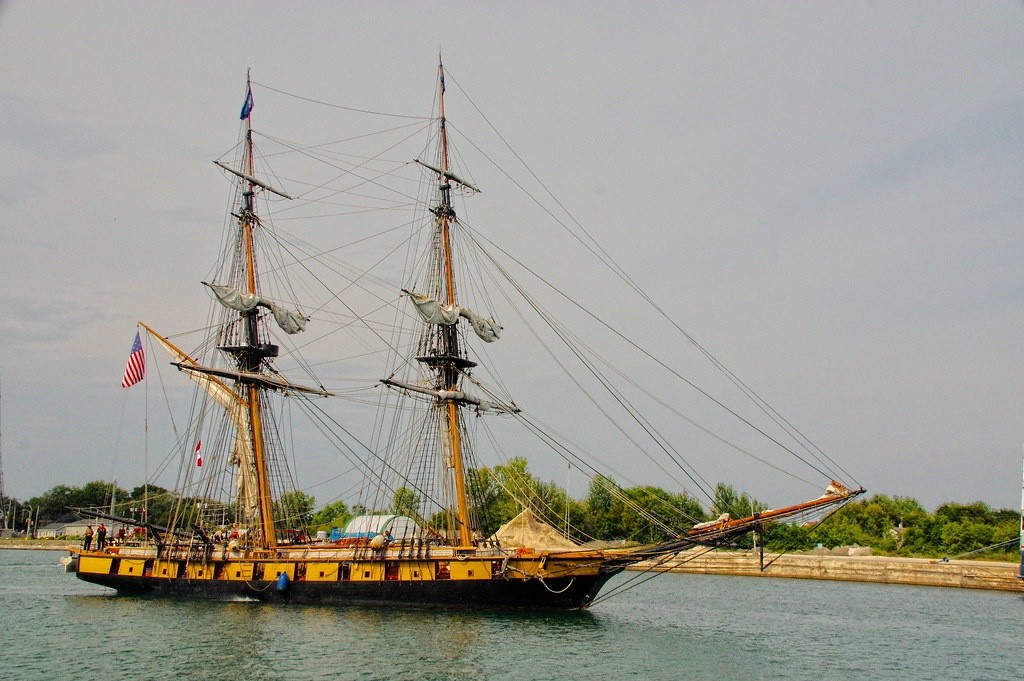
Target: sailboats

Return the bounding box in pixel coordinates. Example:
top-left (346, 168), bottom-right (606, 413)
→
top-left (60, 44), bottom-right (867, 611)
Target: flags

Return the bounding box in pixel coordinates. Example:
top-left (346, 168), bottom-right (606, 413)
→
top-left (121, 331), bottom-right (145, 388)
top-left (196, 440), bottom-right (202, 467)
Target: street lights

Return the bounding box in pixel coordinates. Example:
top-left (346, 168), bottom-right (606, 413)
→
top-left (4, 501), bottom-right (11, 529)
top-left (738, 491), bottom-right (756, 551)
top-left (21, 505), bottom-right (32, 535)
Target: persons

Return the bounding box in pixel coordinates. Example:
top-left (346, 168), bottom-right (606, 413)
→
top-left (84, 523), bottom-right (94, 551)
top-left (97, 523), bottom-right (106, 551)
top-left (214, 527), bottom-right (225, 544)
top-left (385, 531), bottom-right (393, 542)
top-left (229, 530), bottom-right (239, 539)
top-left (471, 528), bottom-right (479, 548)
